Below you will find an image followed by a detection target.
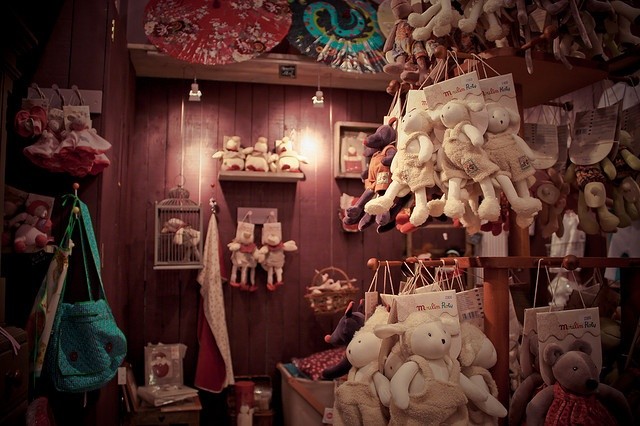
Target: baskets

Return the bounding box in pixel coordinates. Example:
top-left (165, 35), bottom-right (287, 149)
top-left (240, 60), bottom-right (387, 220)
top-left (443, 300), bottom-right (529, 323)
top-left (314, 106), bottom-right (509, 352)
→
top-left (304, 266), bottom-right (362, 316)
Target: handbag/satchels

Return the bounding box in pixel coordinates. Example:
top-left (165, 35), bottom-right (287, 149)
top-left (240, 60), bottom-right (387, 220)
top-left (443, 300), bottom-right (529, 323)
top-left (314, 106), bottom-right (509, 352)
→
top-left (536, 263), bottom-right (604, 387)
top-left (382, 50), bottom-right (523, 139)
top-left (520, 75), bottom-right (640, 171)
top-left (365, 257), bottom-right (486, 336)
top-left (33, 194), bottom-right (128, 394)
top-left (520, 258), bottom-right (566, 376)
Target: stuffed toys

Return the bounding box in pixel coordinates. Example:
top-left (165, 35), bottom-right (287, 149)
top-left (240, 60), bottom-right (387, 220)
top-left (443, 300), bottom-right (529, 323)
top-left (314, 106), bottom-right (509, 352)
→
top-left (343, 98), bottom-right (639, 239)
top-left (22, 110), bottom-right (63, 174)
top-left (50, 109), bottom-right (111, 179)
top-left (226, 225), bottom-right (261, 292)
top-left (10, 199), bottom-right (52, 253)
top-left (507, 327), bottom-right (631, 426)
top-left (371, 1), bottom-right (639, 95)
top-left (211, 133), bottom-right (310, 174)
top-left (334, 302), bottom-right (509, 426)
top-left (258, 228), bottom-right (298, 291)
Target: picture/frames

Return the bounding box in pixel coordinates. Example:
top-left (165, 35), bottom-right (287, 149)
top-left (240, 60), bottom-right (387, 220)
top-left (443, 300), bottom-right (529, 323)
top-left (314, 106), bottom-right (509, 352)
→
top-left (330, 120), bottom-right (383, 181)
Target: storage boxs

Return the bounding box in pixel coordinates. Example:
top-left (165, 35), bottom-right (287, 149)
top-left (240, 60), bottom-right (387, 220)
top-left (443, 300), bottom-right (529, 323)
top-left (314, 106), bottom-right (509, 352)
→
top-left (225, 375), bottom-right (274, 425)
top-left (118, 362), bottom-right (200, 425)
top-left (277, 362), bottom-right (336, 425)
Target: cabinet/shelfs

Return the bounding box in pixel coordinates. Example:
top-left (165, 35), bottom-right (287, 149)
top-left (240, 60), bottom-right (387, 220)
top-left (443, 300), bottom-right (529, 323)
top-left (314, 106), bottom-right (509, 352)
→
top-left (0, 326), bottom-right (30, 425)
top-left (365, 44), bottom-right (640, 424)
